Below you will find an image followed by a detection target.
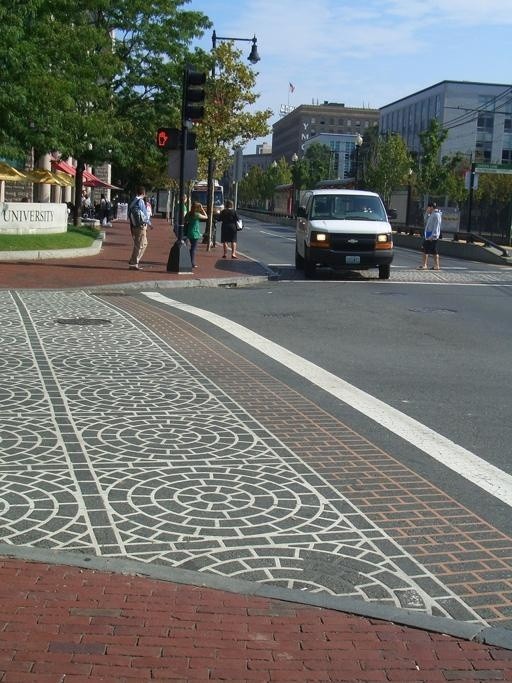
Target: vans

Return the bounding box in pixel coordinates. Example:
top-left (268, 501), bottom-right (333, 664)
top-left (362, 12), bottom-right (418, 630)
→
top-left (294, 188), bottom-right (399, 281)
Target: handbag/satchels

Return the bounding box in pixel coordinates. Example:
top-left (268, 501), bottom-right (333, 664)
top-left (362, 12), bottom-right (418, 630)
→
top-left (235, 219), bottom-right (243, 230)
top-left (183, 223), bottom-right (189, 235)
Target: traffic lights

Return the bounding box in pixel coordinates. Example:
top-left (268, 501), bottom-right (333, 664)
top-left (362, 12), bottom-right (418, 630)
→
top-left (155, 67), bottom-right (206, 153)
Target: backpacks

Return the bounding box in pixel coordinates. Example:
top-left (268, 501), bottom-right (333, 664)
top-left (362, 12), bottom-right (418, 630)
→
top-left (130, 198), bottom-right (145, 227)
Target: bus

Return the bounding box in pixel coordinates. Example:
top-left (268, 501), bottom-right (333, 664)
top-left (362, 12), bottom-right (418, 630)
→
top-left (190, 180), bottom-right (225, 220)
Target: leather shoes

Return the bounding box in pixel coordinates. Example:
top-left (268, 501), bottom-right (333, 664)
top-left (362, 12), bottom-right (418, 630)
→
top-left (128, 263), bottom-right (143, 270)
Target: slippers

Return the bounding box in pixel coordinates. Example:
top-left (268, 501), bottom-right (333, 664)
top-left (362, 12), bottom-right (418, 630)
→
top-left (417, 264), bottom-right (439, 270)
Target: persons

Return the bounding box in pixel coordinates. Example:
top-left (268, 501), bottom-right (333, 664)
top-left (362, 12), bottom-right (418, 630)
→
top-left (184, 201), bottom-right (208, 268)
top-left (418, 201), bottom-right (442, 270)
top-left (175, 193), bottom-right (188, 240)
top-left (128, 186), bottom-right (152, 270)
top-left (217, 200), bottom-right (239, 258)
top-left (82, 191), bottom-right (119, 226)
top-left (145, 195), bottom-right (158, 220)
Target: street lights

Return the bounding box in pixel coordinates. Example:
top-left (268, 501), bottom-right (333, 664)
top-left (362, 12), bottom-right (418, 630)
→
top-left (291, 153), bottom-right (298, 219)
top-left (354, 133), bottom-right (363, 187)
top-left (404, 166), bottom-right (413, 233)
top-left (270, 160), bottom-right (278, 212)
top-left (201, 28), bottom-right (261, 245)
